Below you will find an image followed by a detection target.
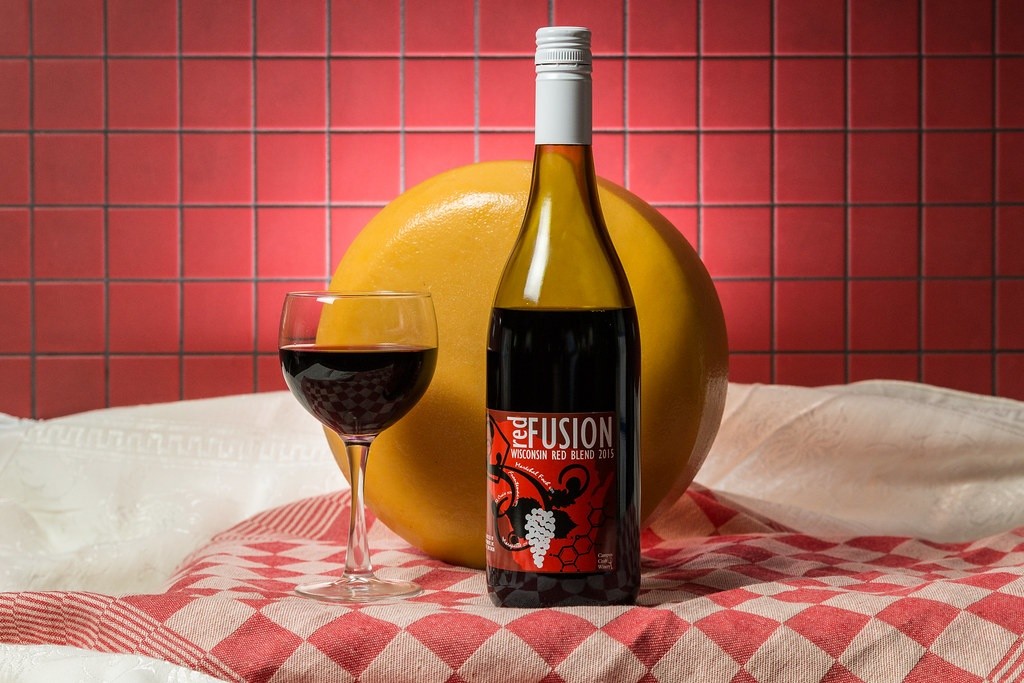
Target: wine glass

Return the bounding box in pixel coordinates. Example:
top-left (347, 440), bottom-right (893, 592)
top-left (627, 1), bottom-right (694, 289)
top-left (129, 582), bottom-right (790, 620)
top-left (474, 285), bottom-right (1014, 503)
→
top-left (278, 290), bottom-right (440, 604)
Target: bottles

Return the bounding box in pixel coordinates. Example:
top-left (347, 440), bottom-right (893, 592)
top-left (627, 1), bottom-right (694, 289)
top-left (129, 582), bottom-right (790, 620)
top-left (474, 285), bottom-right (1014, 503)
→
top-left (484, 27), bottom-right (642, 609)
